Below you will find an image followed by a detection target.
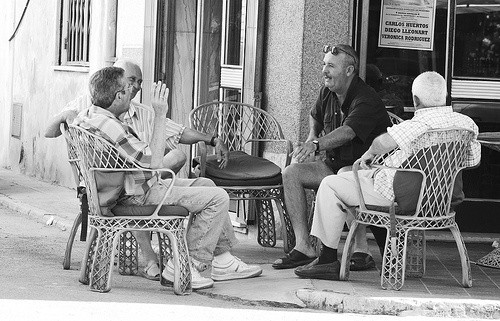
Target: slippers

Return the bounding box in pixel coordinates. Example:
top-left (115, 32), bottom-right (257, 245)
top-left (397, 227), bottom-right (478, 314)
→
top-left (140, 260), bottom-right (160, 280)
top-left (349, 254), bottom-right (375, 269)
top-left (272, 249), bottom-right (318, 269)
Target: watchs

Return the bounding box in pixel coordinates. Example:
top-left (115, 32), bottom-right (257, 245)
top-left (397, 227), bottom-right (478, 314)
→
top-left (313, 140), bottom-right (320, 153)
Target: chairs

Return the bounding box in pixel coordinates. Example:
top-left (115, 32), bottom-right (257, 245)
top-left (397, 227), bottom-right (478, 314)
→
top-left (59, 101), bottom-right (481, 295)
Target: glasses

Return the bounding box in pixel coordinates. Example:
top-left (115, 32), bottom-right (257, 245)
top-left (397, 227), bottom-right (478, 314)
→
top-left (322, 45), bottom-right (357, 64)
top-left (113, 84), bottom-right (134, 100)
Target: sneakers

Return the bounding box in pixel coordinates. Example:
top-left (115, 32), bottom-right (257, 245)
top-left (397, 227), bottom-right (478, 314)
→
top-left (211, 256), bottom-right (263, 280)
top-left (160, 260), bottom-right (215, 290)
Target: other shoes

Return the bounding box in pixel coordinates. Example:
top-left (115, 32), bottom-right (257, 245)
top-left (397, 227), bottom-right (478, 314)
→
top-left (294, 256), bottom-right (340, 279)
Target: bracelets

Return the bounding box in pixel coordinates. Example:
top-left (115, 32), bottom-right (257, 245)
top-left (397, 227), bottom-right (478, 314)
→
top-left (210, 137), bottom-right (216, 147)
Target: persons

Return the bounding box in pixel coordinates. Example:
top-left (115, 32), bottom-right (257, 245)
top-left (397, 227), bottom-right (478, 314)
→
top-left (366, 64), bottom-right (409, 118)
top-left (44, 60), bottom-right (230, 281)
top-left (294, 71), bottom-right (481, 279)
top-left (73, 68), bottom-right (263, 289)
top-left (272, 43), bottom-right (388, 271)
